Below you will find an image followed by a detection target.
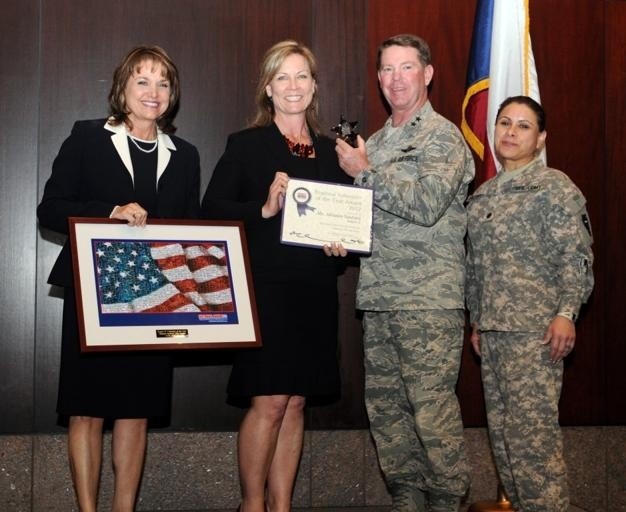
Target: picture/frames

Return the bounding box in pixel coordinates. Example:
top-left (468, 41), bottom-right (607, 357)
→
top-left (67, 214), bottom-right (264, 353)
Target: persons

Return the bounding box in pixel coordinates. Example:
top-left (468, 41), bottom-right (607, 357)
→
top-left (460, 95), bottom-right (594, 510)
top-left (194, 40), bottom-right (359, 511)
top-left (332, 32), bottom-right (478, 512)
top-left (34, 42), bottom-right (203, 506)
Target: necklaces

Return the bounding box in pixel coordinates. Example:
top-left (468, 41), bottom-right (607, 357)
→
top-left (280, 132), bottom-right (318, 160)
top-left (122, 126), bottom-right (159, 155)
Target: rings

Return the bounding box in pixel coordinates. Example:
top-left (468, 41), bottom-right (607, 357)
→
top-left (562, 344), bottom-right (572, 353)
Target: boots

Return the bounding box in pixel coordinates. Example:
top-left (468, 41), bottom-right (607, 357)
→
top-left (385, 487), bottom-right (461, 508)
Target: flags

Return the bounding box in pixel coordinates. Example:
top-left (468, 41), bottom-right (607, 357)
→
top-left (92, 238), bottom-right (238, 316)
top-left (455, 0), bottom-right (549, 186)
